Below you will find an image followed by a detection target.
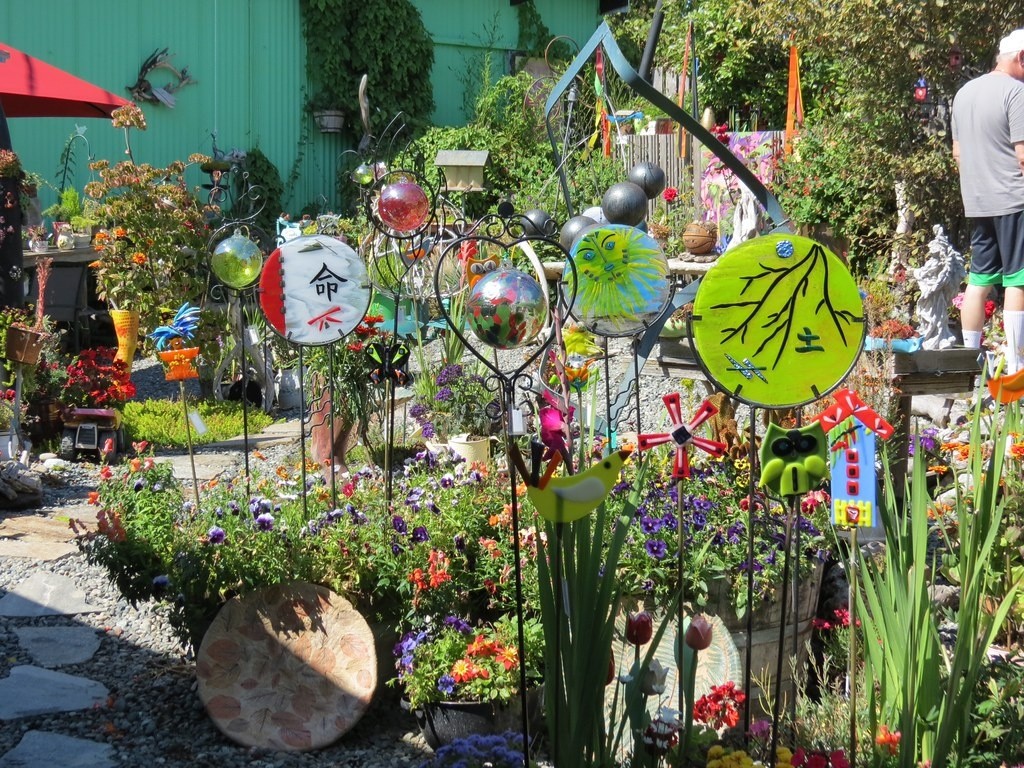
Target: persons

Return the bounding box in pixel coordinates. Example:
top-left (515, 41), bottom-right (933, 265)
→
top-left (951, 27), bottom-right (1024, 375)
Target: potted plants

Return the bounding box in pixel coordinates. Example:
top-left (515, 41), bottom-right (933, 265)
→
top-left (26, 186), bottom-right (92, 253)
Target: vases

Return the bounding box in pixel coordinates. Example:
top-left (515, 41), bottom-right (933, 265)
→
top-left (109, 308), bottom-right (140, 378)
top-left (399, 685), bottom-right (539, 752)
top-left (6, 325), bottom-right (46, 365)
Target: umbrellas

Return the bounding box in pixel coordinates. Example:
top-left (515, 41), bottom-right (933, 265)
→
top-left (0, 43), bottom-right (137, 119)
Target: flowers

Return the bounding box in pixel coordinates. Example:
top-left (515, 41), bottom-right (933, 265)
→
top-left (10, 322), bottom-right (41, 336)
top-left (91, 229), bottom-right (151, 312)
top-left (63, 362), bottom-right (830, 709)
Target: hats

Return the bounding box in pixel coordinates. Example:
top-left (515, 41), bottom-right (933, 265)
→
top-left (1000, 29), bottom-right (1024, 52)
